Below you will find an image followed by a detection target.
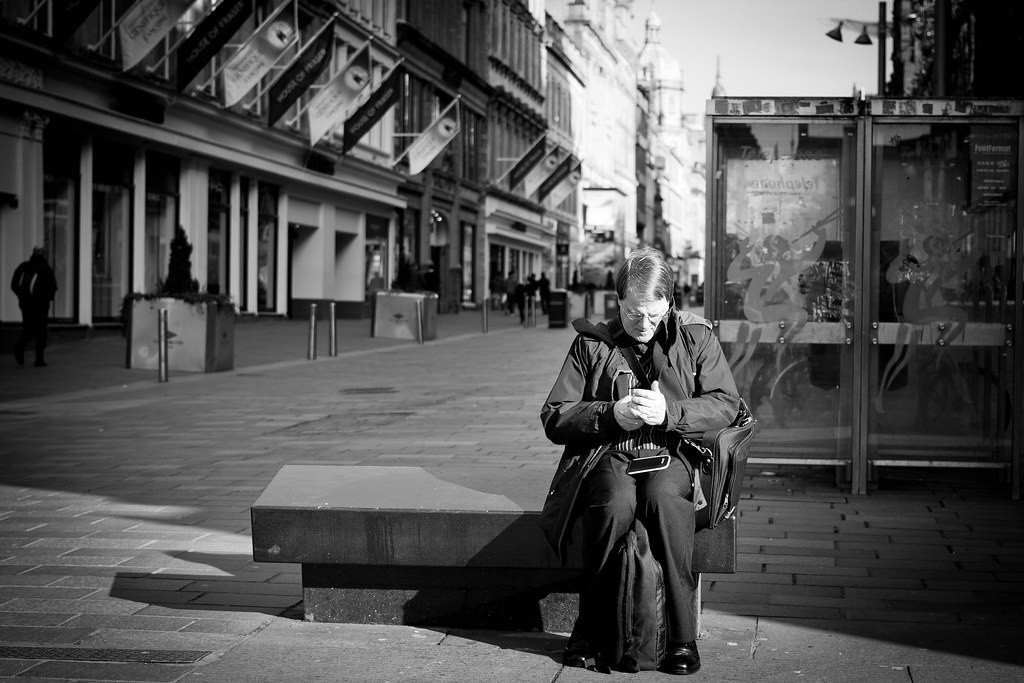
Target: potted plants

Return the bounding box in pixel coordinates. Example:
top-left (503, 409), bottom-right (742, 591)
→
top-left (373, 257), bottom-right (438, 339)
top-left (130, 222), bottom-right (236, 374)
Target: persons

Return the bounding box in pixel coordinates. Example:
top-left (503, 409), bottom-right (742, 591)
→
top-left (540, 249), bottom-right (740, 676)
top-left (502, 269), bottom-right (552, 325)
top-left (11, 246), bottom-right (58, 367)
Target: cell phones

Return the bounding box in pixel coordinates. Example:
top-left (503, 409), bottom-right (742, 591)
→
top-left (627, 456), bottom-right (672, 475)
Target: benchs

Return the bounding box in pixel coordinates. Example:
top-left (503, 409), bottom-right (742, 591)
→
top-left (251, 463), bottom-right (738, 643)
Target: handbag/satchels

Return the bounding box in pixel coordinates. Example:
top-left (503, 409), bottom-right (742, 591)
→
top-left (619, 520), bottom-right (667, 670)
top-left (696, 391), bottom-right (756, 529)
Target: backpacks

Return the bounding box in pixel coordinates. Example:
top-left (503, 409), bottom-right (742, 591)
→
top-left (16, 265), bottom-right (41, 300)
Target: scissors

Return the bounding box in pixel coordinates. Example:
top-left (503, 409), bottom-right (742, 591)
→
top-left (604, 293), bottom-right (620, 320)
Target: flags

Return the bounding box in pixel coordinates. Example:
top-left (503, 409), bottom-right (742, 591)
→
top-left (0, 0), bottom-right (582, 214)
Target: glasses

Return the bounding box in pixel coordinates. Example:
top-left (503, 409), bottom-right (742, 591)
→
top-left (621, 300), bottom-right (670, 321)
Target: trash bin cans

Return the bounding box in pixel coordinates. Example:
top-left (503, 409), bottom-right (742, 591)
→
top-left (547, 290), bottom-right (571, 329)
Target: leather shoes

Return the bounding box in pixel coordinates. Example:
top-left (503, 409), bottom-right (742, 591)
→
top-left (669, 640), bottom-right (700, 674)
top-left (562, 634), bottom-right (591, 668)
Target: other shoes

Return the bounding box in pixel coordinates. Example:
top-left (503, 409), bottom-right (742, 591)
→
top-left (33, 358), bottom-right (48, 365)
top-left (14, 349), bottom-right (25, 364)
